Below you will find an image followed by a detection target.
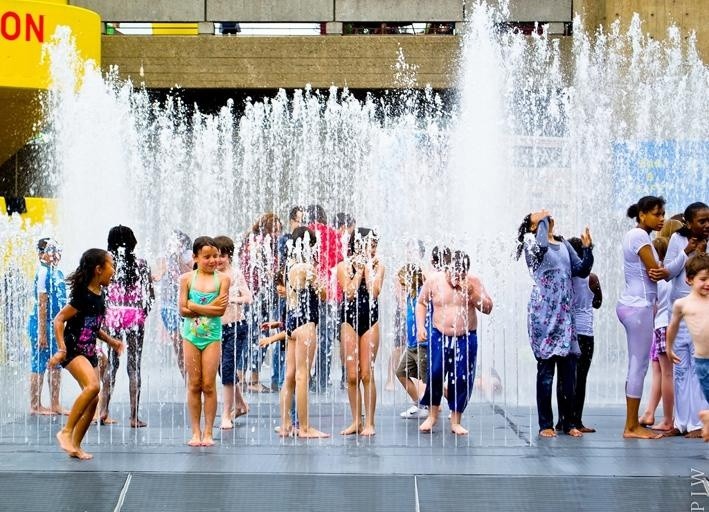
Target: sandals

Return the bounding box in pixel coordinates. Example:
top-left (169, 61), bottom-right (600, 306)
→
top-left (248, 381), bottom-right (274, 393)
top-left (239, 381), bottom-right (247, 393)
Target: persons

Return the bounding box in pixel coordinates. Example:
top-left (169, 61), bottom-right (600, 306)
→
top-left (219, 22), bottom-right (241, 36)
top-left (615, 196), bottom-right (709, 442)
top-left (555, 235), bottom-right (603, 433)
top-left (509, 209), bottom-right (595, 438)
top-left (47, 248), bottom-right (124, 459)
top-left (26, 237), bottom-right (71, 416)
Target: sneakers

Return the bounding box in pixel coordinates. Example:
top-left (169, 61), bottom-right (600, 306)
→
top-left (420, 405), bottom-right (429, 419)
top-left (400, 406), bottom-right (421, 418)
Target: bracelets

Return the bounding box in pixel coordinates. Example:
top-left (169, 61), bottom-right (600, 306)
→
top-left (58, 350), bottom-right (67, 352)
